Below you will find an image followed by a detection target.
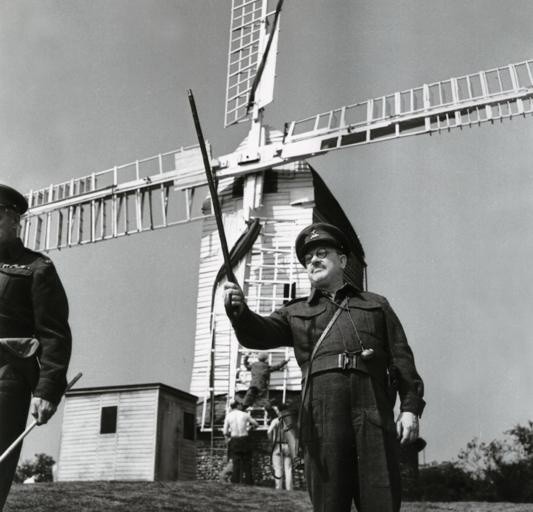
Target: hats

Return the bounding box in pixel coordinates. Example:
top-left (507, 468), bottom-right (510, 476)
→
top-left (295, 223), bottom-right (350, 266)
top-left (0, 184), bottom-right (28, 214)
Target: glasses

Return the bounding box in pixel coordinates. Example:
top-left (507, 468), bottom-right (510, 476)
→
top-left (301, 247), bottom-right (339, 264)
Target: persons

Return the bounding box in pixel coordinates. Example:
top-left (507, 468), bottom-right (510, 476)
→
top-left (222, 222), bottom-right (426, 508)
top-left (1, 183), bottom-right (73, 507)
top-left (223, 351), bottom-right (293, 491)
